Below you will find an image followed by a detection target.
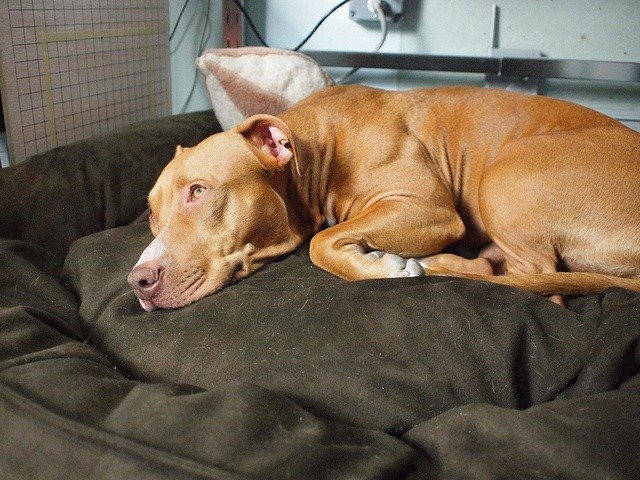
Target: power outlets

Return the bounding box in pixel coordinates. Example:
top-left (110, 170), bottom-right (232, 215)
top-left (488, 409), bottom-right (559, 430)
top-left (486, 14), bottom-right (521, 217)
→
top-left (349, 0), bottom-right (404, 23)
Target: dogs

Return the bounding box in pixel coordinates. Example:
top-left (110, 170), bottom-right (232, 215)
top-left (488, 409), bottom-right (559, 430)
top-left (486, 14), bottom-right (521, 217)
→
top-left (127, 84), bottom-right (640, 313)
